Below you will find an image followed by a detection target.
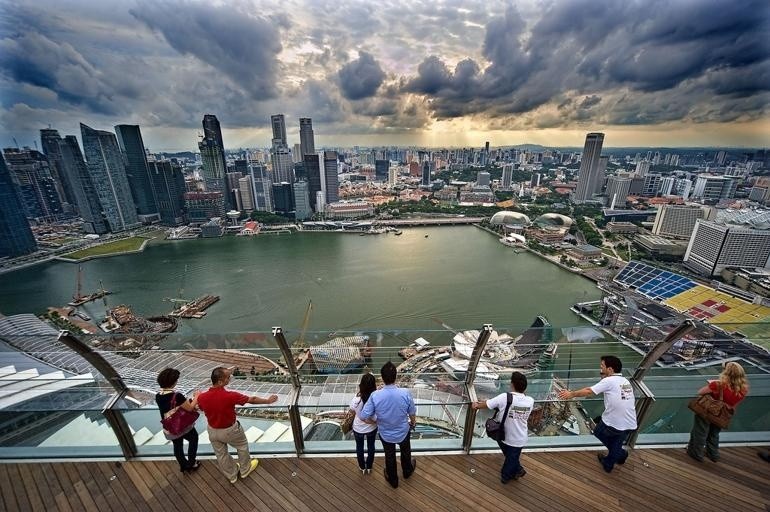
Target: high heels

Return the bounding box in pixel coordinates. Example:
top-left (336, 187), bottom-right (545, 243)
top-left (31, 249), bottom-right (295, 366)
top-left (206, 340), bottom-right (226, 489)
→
top-left (358, 465), bottom-right (371, 475)
top-left (179, 460), bottom-right (188, 476)
top-left (186, 460), bottom-right (201, 476)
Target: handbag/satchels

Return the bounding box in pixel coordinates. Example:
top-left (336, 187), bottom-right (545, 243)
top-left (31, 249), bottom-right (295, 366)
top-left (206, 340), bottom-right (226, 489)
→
top-left (687, 381), bottom-right (736, 430)
top-left (485, 392), bottom-right (513, 442)
top-left (341, 397), bottom-right (361, 436)
top-left (159, 392), bottom-right (200, 437)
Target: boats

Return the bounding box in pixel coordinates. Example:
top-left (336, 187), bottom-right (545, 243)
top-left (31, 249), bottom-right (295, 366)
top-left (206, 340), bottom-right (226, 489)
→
top-left (168, 265), bottom-right (220, 318)
top-left (424, 234), bottom-right (429, 238)
top-left (360, 228), bottom-right (402, 236)
top-left (499, 237), bottom-right (524, 254)
top-left (67, 263), bottom-right (109, 306)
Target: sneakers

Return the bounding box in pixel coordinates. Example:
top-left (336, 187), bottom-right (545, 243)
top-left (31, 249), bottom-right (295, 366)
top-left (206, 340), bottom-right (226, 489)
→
top-left (240, 458), bottom-right (259, 479)
top-left (597, 453), bottom-right (613, 473)
top-left (686, 445), bottom-right (718, 463)
top-left (617, 450), bottom-right (628, 465)
top-left (501, 466), bottom-right (526, 484)
top-left (229, 462), bottom-right (240, 484)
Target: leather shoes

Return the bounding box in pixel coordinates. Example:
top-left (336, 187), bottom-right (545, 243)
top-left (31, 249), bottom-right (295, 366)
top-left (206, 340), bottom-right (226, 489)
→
top-left (403, 458), bottom-right (416, 479)
top-left (384, 468), bottom-right (399, 489)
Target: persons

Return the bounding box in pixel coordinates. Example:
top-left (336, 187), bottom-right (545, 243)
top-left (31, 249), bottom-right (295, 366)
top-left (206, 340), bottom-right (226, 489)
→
top-left (558, 357), bottom-right (651, 473)
top-left (155, 367), bottom-right (278, 484)
top-left (470, 370), bottom-right (542, 484)
top-left (687, 358), bottom-right (749, 462)
top-left (341, 360), bottom-right (419, 489)
